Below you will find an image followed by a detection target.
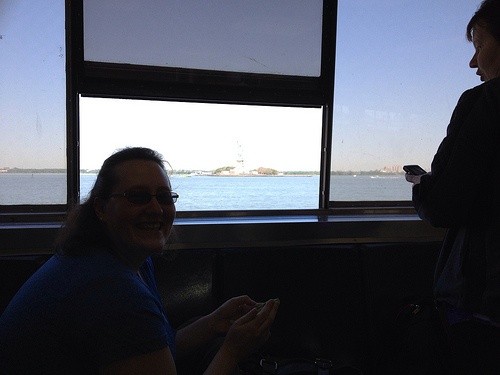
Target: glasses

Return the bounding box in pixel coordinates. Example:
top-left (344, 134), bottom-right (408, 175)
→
top-left (105, 190), bottom-right (179, 206)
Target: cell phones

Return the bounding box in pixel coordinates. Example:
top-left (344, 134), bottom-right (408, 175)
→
top-left (403, 165), bottom-right (427, 176)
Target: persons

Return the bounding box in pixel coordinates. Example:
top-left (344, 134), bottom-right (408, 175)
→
top-left (1, 148), bottom-right (279, 375)
top-left (406, 0), bottom-right (500, 375)
